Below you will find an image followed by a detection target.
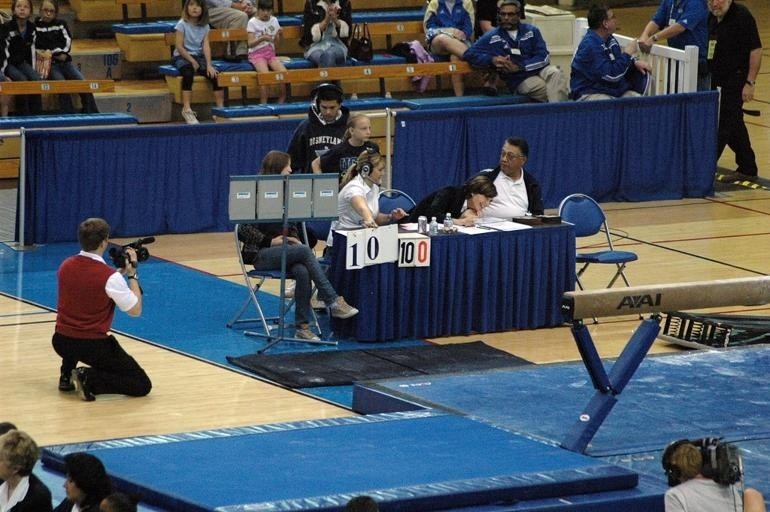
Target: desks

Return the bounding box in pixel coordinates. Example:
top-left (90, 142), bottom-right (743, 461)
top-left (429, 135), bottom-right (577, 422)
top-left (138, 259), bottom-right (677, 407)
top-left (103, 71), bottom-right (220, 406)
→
top-left (331, 216), bottom-right (577, 344)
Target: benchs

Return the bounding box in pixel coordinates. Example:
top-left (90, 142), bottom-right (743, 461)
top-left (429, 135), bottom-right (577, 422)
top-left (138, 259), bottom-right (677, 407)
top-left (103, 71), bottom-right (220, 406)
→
top-left (107, 1), bottom-right (533, 124)
top-left (2, 1), bottom-right (117, 137)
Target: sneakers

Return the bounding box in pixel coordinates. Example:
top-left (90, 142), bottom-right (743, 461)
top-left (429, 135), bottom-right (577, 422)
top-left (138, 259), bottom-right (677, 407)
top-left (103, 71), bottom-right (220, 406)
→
top-left (294, 328), bottom-right (320, 340)
top-left (72, 368), bottom-right (95, 401)
top-left (182, 106), bottom-right (200, 125)
top-left (720, 170), bottom-right (757, 183)
top-left (60, 363), bottom-right (77, 391)
top-left (331, 296), bottom-right (359, 318)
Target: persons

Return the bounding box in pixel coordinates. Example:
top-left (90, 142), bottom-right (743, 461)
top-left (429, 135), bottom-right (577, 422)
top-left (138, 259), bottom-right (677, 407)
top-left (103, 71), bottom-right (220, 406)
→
top-left (34, 0), bottom-right (99, 114)
top-left (0, 0), bottom-right (43, 116)
top-left (347, 495), bottom-right (380, 512)
top-left (0, 421), bottom-right (146, 512)
top-left (663, 441), bottom-right (766, 512)
top-left (52, 218), bottom-right (151, 401)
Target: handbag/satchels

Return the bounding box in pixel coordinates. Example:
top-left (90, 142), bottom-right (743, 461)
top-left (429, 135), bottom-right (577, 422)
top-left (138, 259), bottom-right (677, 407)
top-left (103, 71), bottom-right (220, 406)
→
top-left (349, 36), bottom-right (372, 62)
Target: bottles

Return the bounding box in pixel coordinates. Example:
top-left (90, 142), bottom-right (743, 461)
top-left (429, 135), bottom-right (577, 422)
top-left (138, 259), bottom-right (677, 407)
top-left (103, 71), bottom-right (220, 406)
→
top-left (444, 213), bottom-right (454, 233)
top-left (429, 217), bottom-right (438, 235)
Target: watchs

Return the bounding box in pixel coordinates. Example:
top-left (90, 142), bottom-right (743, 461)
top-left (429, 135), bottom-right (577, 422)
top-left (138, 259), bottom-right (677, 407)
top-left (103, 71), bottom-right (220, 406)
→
top-left (128, 273), bottom-right (138, 279)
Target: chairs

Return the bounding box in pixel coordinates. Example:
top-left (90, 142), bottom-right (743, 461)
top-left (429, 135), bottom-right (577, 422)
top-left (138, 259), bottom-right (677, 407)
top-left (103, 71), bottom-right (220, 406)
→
top-left (226, 188), bottom-right (418, 342)
top-left (560, 193), bottom-right (644, 324)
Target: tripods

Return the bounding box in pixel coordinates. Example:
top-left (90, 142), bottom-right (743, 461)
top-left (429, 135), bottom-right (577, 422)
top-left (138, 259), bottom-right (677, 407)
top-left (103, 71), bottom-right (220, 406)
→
top-left (360, 143), bottom-right (376, 178)
top-left (241, 235), bottom-right (341, 357)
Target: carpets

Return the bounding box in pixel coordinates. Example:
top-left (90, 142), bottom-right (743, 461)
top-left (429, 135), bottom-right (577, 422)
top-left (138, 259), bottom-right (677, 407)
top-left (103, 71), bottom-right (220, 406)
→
top-left (222, 339), bottom-right (537, 388)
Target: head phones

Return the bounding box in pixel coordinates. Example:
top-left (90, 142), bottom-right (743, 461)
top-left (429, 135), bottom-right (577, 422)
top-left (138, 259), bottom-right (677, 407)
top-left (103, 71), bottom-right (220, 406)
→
top-left (662, 441), bottom-right (682, 485)
